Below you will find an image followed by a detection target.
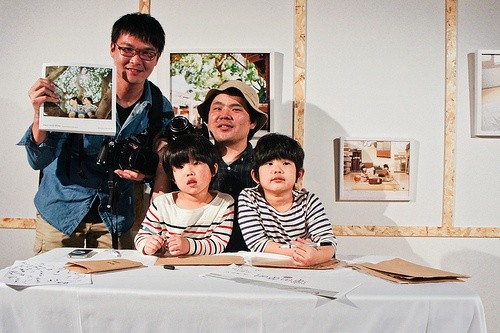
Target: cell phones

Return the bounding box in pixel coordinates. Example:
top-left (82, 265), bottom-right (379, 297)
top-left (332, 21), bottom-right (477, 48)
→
top-left (67, 249), bottom-right (93, 259)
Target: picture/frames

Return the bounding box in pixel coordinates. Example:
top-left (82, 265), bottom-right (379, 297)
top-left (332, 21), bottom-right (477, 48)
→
top-left (167, 46), bottom-right (274, 137)
top-left (337, 137), bottom-right (415, 201)
top-left (472, 49), bottom-right (500, 137)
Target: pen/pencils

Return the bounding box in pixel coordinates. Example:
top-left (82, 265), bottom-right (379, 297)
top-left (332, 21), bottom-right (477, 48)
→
top-left (145, 227), bottom-right (164, 240)
top-left (112, 249), bottom-right (120, 257)
top-left (289, 242), bottom-right (332, 248)
top-left (279, 245), bottom-right (289, 248)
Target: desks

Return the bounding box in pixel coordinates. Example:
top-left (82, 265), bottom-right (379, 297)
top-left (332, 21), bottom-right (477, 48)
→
top-left (1, 247), bottom-right (487, 333)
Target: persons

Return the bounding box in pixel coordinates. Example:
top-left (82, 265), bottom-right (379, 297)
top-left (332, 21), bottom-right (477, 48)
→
top-left (150, 81), bottom-right (270, 251)
top-left (20, 13), bottom-right (174, 254)
top-left (238, 133), bottom-right (337, 266)
top-left (134, 133), bottom-right (234, 257)
top-left (361, 163), bottom-right (390, 181)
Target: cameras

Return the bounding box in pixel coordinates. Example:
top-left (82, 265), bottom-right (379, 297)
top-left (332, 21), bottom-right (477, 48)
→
top-left (165, 116), bottom-right (197, 143)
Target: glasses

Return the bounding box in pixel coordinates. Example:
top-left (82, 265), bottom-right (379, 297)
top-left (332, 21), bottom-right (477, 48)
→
top-left (115, 41), bottom-right (160, 61)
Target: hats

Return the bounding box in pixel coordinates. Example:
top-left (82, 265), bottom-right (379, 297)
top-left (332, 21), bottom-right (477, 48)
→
top-left (197, 80), bottom-right (268, 141)
